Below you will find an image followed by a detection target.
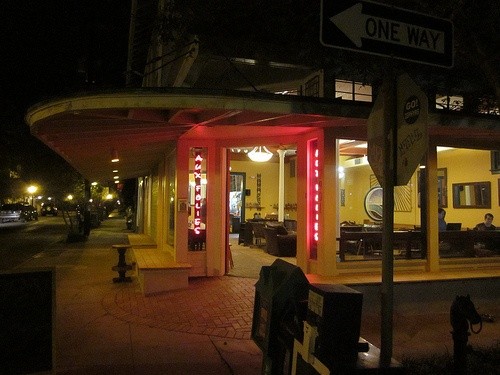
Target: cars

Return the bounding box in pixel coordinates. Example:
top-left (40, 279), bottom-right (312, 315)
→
top-left (41, 201), bottom-right (58, 216)
top-left (0, 202), bottom-right (38, 224)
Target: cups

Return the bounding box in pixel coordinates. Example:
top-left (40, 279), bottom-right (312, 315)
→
top-left (478, 226), bottom-right (484, 231)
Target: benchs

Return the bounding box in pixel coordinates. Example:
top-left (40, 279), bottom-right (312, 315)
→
top-left (251, 221), bottom-right (267, 245)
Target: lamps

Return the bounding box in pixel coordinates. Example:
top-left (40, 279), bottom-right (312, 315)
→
top-left (248, 145), bottom-right (273, 162)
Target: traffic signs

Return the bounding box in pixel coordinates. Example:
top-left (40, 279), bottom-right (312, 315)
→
top-left (320, 0), bottom-right (456, 71)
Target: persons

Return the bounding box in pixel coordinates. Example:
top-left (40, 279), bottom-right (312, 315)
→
top-left (438, 208), bottom-right (447, 230)
top-left (476, 213), bottom-right (496, 230)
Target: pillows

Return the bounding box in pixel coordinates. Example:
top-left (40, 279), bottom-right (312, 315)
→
top-left (266, 224), bottom-right (288, 234)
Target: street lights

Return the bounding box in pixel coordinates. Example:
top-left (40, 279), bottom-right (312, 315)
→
top-left (28, 185), bottom-right (36, 207)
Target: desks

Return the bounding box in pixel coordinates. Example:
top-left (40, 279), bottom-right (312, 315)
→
top-left (338, 222), bottom-right (500, 262)
top-left (188, 221), bottom-right (206, 251)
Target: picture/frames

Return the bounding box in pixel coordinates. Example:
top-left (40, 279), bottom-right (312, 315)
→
top-left (417, 168), bottom-right (447, 208)
top-left (452, 181), bottom-right (491, 209)
top-left (363, 186), bottom-right (383, 221)
top-left (369, 174), bottom-right (412, 212)
top-left (490, 150), bottom-right (500, 175)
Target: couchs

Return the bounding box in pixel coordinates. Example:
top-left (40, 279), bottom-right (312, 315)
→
top-left (264, 222), bottom-right (297, 257)
